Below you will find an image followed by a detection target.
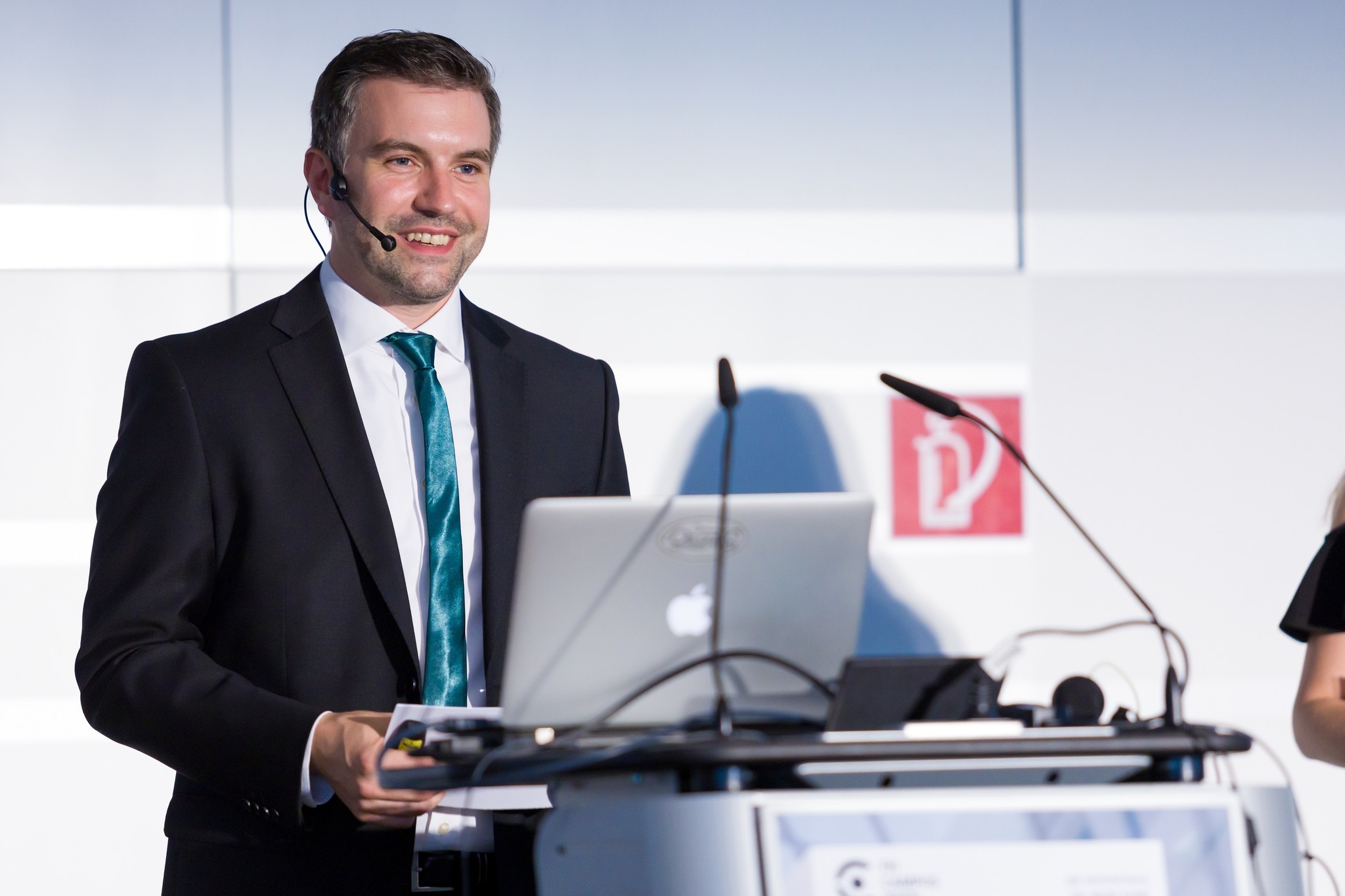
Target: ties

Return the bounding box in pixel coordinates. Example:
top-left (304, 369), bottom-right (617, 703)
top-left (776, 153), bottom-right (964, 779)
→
top-left (381, 331), bottom-right (468, 707)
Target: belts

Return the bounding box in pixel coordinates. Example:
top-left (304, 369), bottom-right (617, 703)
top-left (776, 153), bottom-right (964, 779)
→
top-left (410, 847), bottom-right (533, 892)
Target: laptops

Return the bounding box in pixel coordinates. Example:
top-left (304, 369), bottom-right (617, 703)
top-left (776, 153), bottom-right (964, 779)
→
top-left (478, 491), bottom-right (873, 736)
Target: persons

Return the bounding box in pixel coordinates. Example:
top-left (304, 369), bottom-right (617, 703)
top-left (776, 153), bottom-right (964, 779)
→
top-left (1279, 470), bottom-right (1345, 768)
top-left (75, 29), bottom-right (631, 896)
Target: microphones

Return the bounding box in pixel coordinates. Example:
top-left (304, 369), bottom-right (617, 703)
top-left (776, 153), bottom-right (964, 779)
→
top-left (1050, 676), bottom-right (1104, 725)
top-left (679, 353), bottom-right (767, 745)
top-left (328, 174), bottom-right (396, 252)
top-left (880, 372), bottom-right (1186, 734)
